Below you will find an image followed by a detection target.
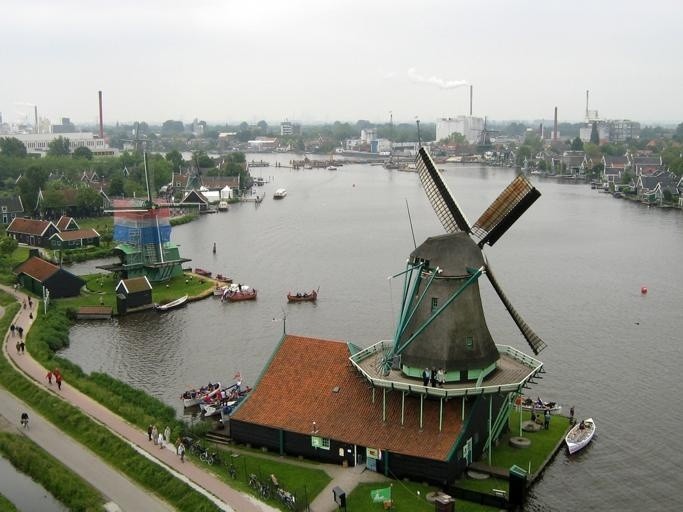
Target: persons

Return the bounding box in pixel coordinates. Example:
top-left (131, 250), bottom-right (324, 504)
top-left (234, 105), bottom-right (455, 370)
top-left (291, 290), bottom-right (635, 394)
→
top-left (148, 425), bottom-right (186, 462)
top-left (516, 396), bottom-right (586, 431)
top-left (183, 381), bottom-right (251, 406)
top-left (46, 369), bottom-right (63, 389)
top-left (10, 283), bottom-right (34, 354)
top-left (21, 412), bottom-right (28, 428)
top-left (422, 367), bottom-right (445, 389)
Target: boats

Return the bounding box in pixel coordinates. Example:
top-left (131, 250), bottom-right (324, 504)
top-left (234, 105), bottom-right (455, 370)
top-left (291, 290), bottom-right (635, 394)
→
top-left (204, 386), bottom-right (253, 417)
top-left (194, 267), bottom-right (213, 277)
top-left (215, 274), bottom-right (233, 285)
top-left (272, 186), bottom-right (288, 200)
top-left (217, 200), bottom-right (229, 212)
top-left (180, 380), bottom-right (222, 409)
top-left (563, 414), bottom-right (597, 458)
top-left (327, 166), bottom-right (338, 171)
top-left (222, 287), bottom-right (257, 302)
top-left (199, 376), bottom-right (243, 412)
top-left (514, 393), bottom-right (563, 417)
top-left (287, 290), bottom-right (318, 303)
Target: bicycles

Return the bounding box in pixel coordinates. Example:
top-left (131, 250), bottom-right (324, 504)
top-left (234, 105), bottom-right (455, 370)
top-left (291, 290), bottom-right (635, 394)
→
top-left (188, 438), bottom-right (297, 511)
